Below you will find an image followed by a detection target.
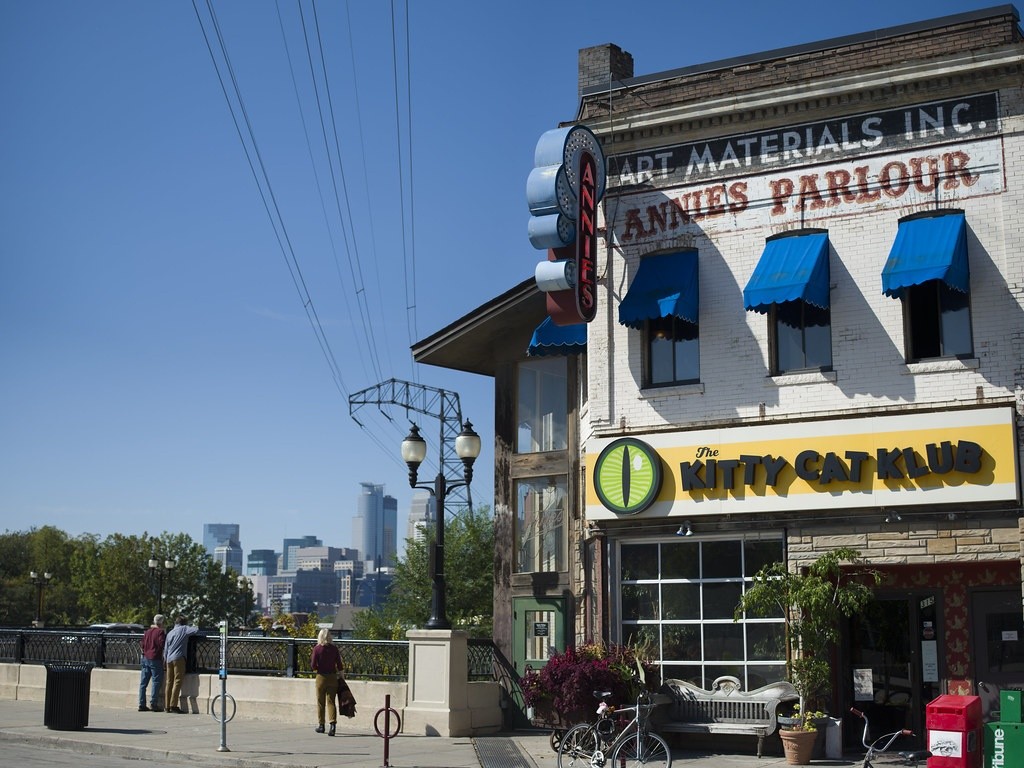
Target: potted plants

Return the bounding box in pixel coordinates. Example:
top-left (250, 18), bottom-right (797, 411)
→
top-left (732, 545), bottom-right (882, 765)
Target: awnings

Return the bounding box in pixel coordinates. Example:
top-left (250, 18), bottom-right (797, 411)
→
top-left (526, 316), bottom-right (587, 359)
top-left (743, 233), bottom-right (830, 315)
top-left (880, 212), bottom-right (971, 300)
top-left (618, 250), bottom-right (699, 330)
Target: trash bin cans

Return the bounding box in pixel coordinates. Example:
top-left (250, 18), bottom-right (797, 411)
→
top-left (42, 659), bottom-right (95, 731)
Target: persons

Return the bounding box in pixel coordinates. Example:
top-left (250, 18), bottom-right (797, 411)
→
top-left (162, 614), bottom-right (197, 714)
top-left (138, 613), bottom-right (166, 712)
top-left (310, 628), bottom-right (345, 736)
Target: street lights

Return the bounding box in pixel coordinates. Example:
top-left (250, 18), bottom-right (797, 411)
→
top-left (148, 554), bottom-right (175, 614)
top-left (30, 568), bottom-right (52, 621)
top-left (401, 417), bottom-right (481, 630)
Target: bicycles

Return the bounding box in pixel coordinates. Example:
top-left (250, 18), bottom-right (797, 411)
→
top-left (557, 678), bottom-right (673, 768)
top-left (850, 707), bottom-right (934, 768)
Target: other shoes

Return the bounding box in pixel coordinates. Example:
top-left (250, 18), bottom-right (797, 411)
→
top-left (164, 708), bottom-right (169, 713)
top-left (315, 724), bottom-right (323, 732)
top-left (328, 723), bottom-right (337, 736)
top-left (138, 705), bottom-right (149, 711)
top-left (170, 707), bottom-right (182, 714)
top-left (150, 705), bottom-right (162, 711)
